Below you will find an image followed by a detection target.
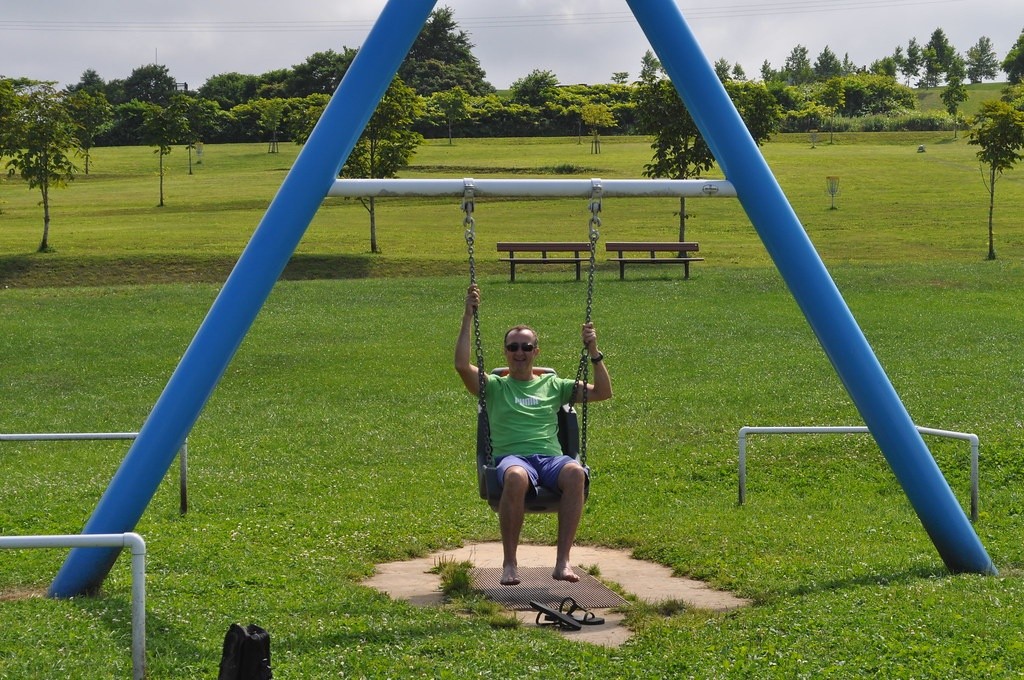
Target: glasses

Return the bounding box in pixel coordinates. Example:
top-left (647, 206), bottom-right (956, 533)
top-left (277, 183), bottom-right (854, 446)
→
top-left (506, 342), bottom-right (536, 353)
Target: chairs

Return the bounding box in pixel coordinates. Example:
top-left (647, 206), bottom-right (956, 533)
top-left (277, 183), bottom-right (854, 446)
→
top-left (471, 363), bottom-right (592, 509)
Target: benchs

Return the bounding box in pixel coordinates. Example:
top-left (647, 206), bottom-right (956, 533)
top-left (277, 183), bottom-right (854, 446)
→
top-left (604, 240), bottom-right (705, 278)
top-left (496, 239), bottom-right (593, 279)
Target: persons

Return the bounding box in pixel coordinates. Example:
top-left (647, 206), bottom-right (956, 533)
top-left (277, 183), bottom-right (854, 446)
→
top-left (456, 283), bottom-right (613, 585)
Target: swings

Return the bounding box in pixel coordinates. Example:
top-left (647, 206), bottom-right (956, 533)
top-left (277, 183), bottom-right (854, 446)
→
top-left (459, 177), bottom-right (602, 513)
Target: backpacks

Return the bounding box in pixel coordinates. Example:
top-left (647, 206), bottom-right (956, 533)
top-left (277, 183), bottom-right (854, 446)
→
top-left (217, 623), bottom-right (272, 680)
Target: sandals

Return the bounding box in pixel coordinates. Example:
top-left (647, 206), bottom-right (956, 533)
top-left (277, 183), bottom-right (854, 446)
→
top-left (530, 601), bottom-right (582, 631)
top-left (559, 597), bottom-right (605, 625)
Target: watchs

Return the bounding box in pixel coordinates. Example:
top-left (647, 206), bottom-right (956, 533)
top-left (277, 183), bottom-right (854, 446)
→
top-left (590, 351), bottom-right (604, 365)
